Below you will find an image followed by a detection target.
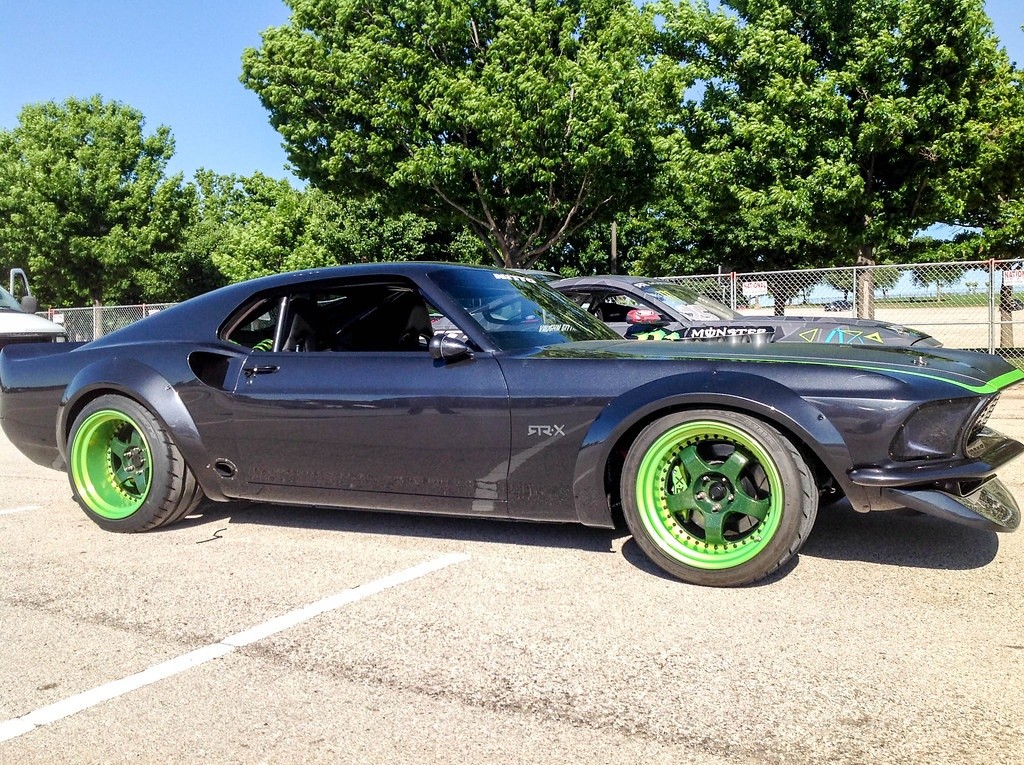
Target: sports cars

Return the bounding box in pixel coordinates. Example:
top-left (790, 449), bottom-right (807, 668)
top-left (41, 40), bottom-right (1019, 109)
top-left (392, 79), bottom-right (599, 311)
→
top-left (428, 274), bottom-right (943, 350)
top-left (0, 256), bottom-right (1023, 591)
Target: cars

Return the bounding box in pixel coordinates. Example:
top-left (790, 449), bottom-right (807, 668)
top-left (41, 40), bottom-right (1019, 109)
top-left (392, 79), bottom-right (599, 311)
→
top-left (0, 284), bottom-right (69, 350)
